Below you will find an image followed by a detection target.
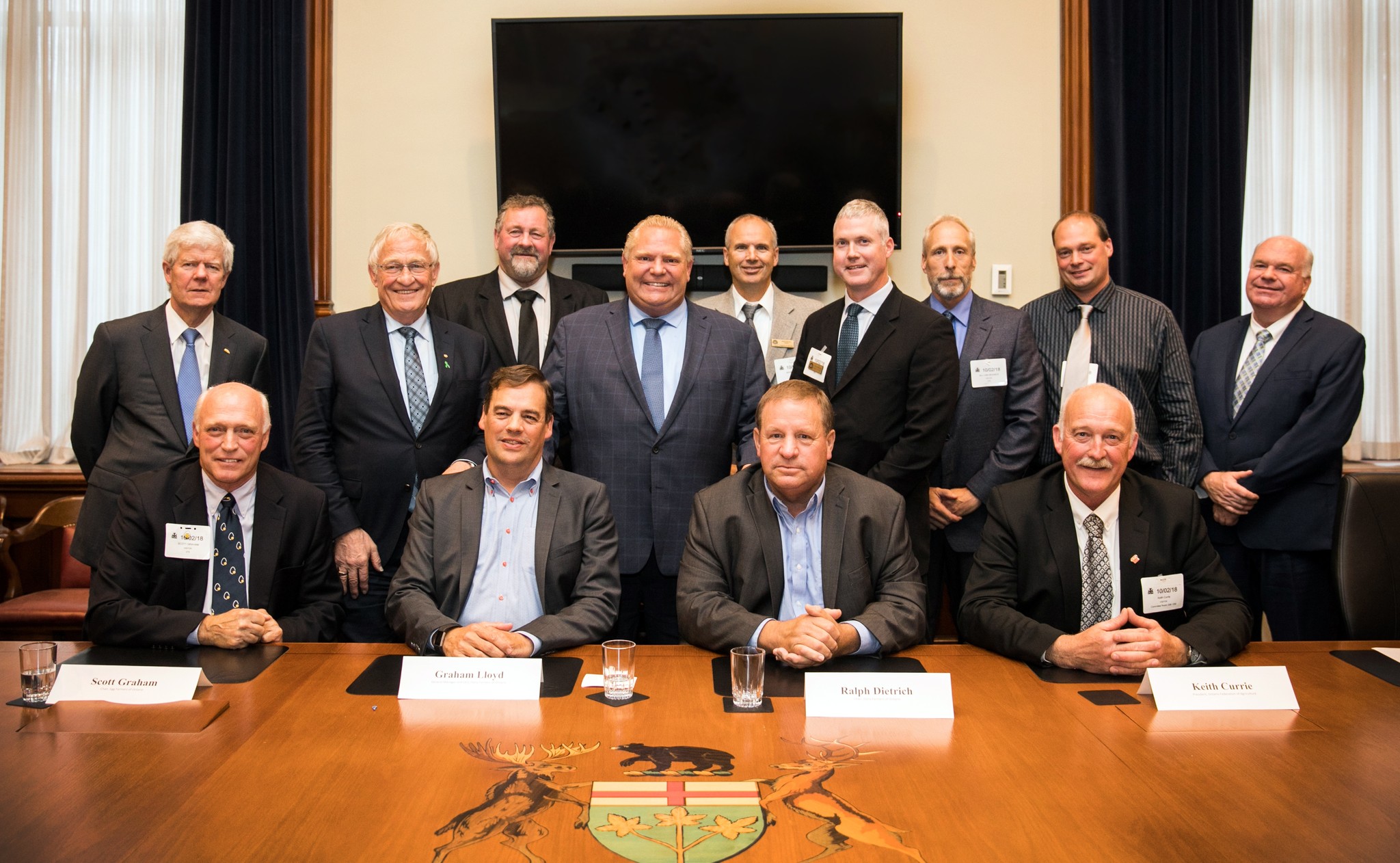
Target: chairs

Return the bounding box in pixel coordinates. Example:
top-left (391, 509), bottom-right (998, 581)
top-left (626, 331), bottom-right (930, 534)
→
top-left (0, 495), bottom-right (91, 641)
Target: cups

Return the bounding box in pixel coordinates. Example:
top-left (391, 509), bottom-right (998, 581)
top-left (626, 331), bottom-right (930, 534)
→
top-left (730, 646), bottom-right (766, 709)
top-left (18, 641), bottom-right (58, 702)
top-left (602, 640), bottom-right (636, 700)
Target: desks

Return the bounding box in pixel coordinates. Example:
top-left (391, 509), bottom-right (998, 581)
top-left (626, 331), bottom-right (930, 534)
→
top-left (0, 636), bottom-right (1400, 863)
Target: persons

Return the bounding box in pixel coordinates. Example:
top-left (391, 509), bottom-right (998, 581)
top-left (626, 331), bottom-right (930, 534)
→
top-left (69, 222), bottom-right (284, 642)
top-left (1189, 235), bottom-right (1366, 640)
top-left (1019, 212), bottom-right (1203, 488)
top-left (920, 212), bottom-right (1043, 637)
top-left (425, 194), bottom-right (609, 379)
top-left (281, 225), bottom-right (488, 645)
top-left (385, 365), bottom-right (622, 659)
top-left (955, 384), bottom-right (1250, 674)
top-left (790, 199), bottom-right (960, 578)
top-left (694, 214), bottom-right (806, 387)
top-left (677, 379), bottom-right (925, 668)
top-left (84, 382), bottom-right (345, 648)
top-left (442, 213), bottom-right (771, 645)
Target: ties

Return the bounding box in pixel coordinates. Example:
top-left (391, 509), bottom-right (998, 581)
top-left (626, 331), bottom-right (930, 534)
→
top-left (211, 493), bottom-right (247, 617)
top-left (397, 327), bottom-right (431, 489)
top-left (742, 303), bottom-right (762, 334)
top-left (514, 290), bottom-right (541, 371)
top-left (835, 304), bottom-right (864, 388)
top-left (942, 310), bottom-right (954, 321)
top-left (1232, 330), bottom-right (1273, 418)
top-left (176, 328), bottom-right (203, 445)
top-left (642, 318), bottom-right (665, 434)
top-left (1060, 305), bottom-right (1095, 414)
top-left (1081, 515), bottom-right (1113, 631)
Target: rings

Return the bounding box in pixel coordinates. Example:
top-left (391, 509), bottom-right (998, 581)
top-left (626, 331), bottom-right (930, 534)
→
top-left (338, 571), bottom-right (347, 576)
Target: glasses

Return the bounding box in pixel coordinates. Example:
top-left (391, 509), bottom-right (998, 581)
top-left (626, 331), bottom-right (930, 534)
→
top-left (377, 262), bottom-right (432, 276)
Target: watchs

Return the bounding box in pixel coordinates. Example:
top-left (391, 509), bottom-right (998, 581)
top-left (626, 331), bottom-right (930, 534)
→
top-left (434, 624), bottom-right (463, 657)
top-left (1187, 644), bottom-right (1199, 664)
